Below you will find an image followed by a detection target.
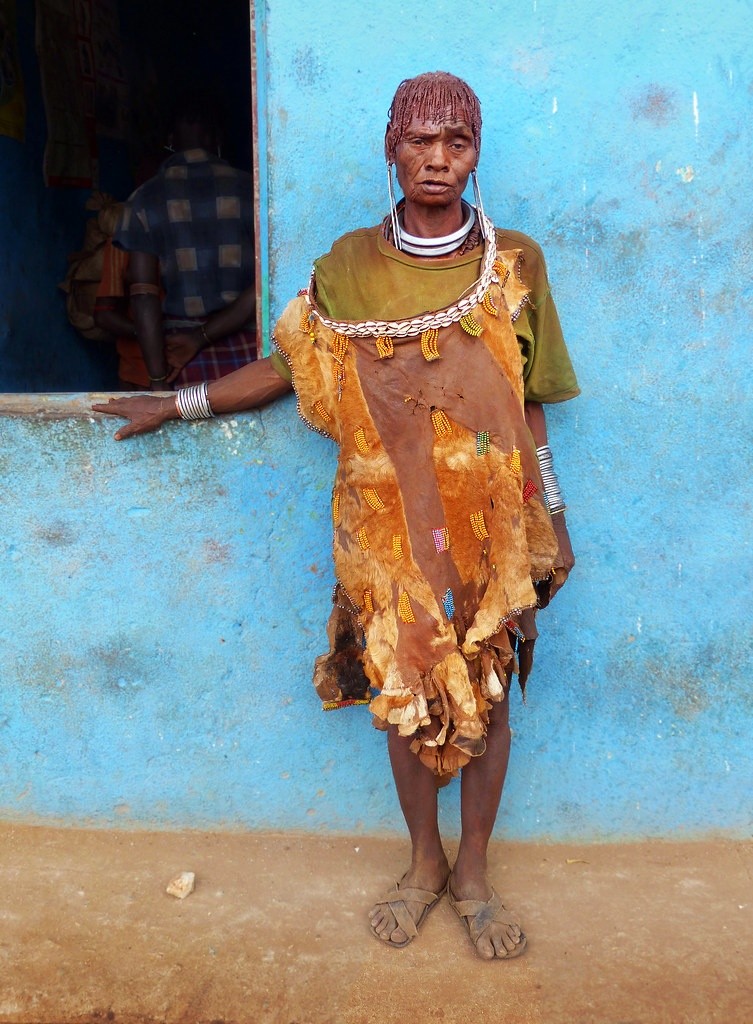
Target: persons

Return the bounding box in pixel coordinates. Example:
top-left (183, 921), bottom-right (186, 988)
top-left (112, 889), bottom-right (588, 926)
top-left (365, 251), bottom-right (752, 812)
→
top-left (60, 92), bottom-right (256, 390)
top-left (91, 71), bottom-right (581, 961)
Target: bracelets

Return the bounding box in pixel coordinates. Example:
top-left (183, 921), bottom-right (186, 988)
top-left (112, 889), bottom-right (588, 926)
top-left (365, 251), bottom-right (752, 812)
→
top-left (130, 283), bottom-right (161, 296)
top-left (536, 445), bottom-right (567, 515)
top-left (148, 371), bottom-right (169, 381)
top-left (174, 381), bottom-right (216, 421)
top-left (94, 305), bottom-right (118, 311)
top-left (201, 325), bottom-right (213, 344)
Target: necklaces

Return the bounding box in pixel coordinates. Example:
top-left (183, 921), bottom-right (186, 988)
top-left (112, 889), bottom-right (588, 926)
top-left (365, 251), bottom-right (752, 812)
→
top-left (392, 200), bottom-right (475, 257)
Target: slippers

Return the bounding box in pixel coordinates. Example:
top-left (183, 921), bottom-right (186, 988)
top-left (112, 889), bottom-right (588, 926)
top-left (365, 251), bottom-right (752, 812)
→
top-left (369, 866), bottom-right (452, 947)
top-left (446, 873), bottom-right (527, 959)
